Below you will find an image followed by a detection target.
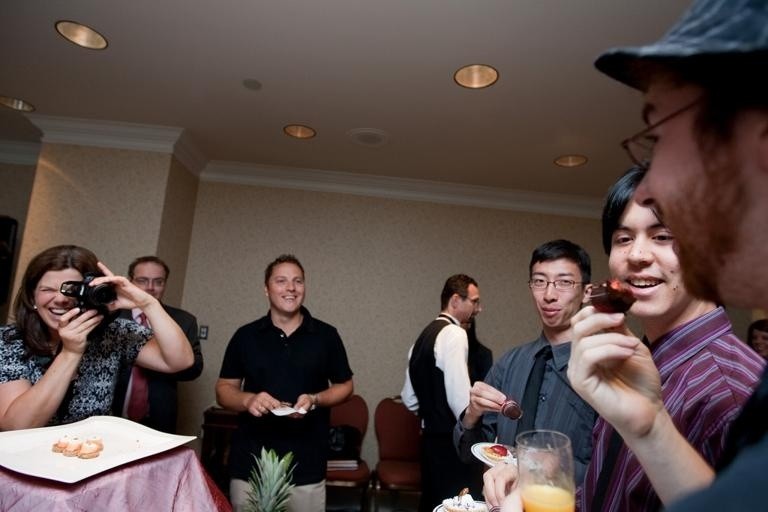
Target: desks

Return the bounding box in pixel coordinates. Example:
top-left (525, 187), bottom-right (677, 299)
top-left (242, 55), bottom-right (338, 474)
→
top-left (0, 443), bottom-right (237, 512)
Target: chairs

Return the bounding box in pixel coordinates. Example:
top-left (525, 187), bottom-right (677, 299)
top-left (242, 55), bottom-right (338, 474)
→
top-left (325, 393), bottom-right (429, 512)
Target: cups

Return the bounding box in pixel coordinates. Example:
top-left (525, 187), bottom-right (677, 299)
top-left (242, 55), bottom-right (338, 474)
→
top-left (512, 425), bottom-right (576, 512)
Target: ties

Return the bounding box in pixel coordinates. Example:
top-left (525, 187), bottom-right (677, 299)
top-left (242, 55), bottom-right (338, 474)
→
top-left (593, 430), bottom-right (625, 511)
top-left (139, 312), bottom-right (150, 328)
top-left (514, 345), bottom-right (553, 446)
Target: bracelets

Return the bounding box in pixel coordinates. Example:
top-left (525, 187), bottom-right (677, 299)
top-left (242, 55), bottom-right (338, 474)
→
top-left (310, 393), bottom-right (317, 413)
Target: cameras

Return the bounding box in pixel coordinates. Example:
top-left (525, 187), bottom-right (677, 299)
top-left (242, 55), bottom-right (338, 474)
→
top-left (60, 272), bottom-right (122, 330)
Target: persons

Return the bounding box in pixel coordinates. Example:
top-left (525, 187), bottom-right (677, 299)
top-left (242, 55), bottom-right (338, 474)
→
top-left (111, 253), bottom-right (205, 432)
top-left (746, 318), bottom-right (767, 354)
top-left (0, 243), bottom-right (196, 433)
top-left (214, 250), bottom-right (354, 512)
top-left (401, 274), bottom-right (485, 512)
top-left (466, 314), bottom-right (493, 386)
top-left (453, 240), bottom-right (598, 487)
top-left (565, 0), bottom-right (767, 512)
top-left (473, 158), bottom-right (767, 509)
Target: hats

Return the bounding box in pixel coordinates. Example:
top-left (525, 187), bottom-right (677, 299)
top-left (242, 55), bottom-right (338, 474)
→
top-left (593, 2), bottom-right (768, 92)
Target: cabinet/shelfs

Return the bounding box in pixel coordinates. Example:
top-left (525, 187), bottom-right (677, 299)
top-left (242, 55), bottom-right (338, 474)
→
top-left (200, 403), bottom-right (247, 501)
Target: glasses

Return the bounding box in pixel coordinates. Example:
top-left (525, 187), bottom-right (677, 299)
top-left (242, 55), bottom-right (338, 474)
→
top-left (136, 278), bottom-right (164, 284)
top-left (527, 279), bottom-right (587, 288)
top-left (621, 90), bottom-right (714, 171)
top-left (466, 296), bottom-right (481, 304)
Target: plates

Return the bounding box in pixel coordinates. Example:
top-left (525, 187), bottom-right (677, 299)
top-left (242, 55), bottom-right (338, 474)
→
top-left (430, 498), bottom-right (488, 512)
top-left (470, 440), bottom-right (517, 470)
top-left (0, 413), bottom-right (197, 486)
